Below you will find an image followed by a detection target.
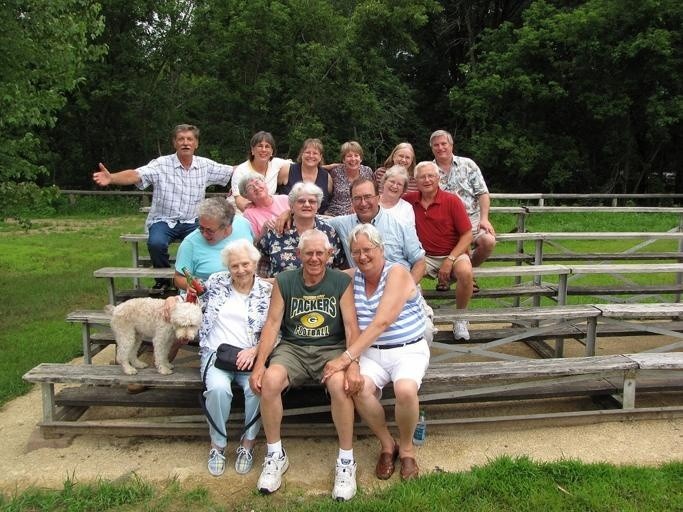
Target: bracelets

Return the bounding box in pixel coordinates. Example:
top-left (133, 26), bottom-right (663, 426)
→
top-left (344, 350), bottom-right (352, 363)
top-left (447, 254), bottom-right (455, 262)
top-left (354, 358), bottom-right (359, 367)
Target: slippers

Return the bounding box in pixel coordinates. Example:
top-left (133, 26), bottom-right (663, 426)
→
top-left (436, 280), bottom-right (450, 291)
top-left (472, 278), bottom-right (479, 292)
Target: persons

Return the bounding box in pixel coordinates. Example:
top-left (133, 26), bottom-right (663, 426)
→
top-left (347, 161), bottom-right (474, 341)
top-left (225, 131), bottom-right (344, 215)
top-left (255, 182), bottom-right (346, 278)
top-left (171, 196), bottom-right (255, 296)
top-left (246, 228), bottom-right (359, 502)
top-left (376, 164), bottom-right (416, 233)
top-left (238, 172), bottom-right (290, 239)
top-left (276, 136), bottom-right (333, 215)
top-left (324, 139), bottom-right (375, 217)
top-left (274, 176), bottom-right (427, 286)
top-left (429, 128), bottom-right (497, 293)
top-left (92, 123), bottom-right (236, 297)
top-left (172, 238), bottom-right (282, 479)
top-left (373, 141), bottom-right (420, 197)
top-left (261, 222), bottom-right (431, 488)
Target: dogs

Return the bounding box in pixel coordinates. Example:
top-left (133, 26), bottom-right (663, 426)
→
top-left (103, 294), bottom-right (208, 376)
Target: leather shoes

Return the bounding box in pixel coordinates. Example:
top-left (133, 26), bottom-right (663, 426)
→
top-left (376, 440), bottom-right (398, 479)
top-left (400, 457), bottom-right (418, 482)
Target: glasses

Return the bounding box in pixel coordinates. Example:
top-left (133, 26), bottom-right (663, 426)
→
top-left (198, 224), bottom-right (222, 234)
top-left (350, 244), bottom-right (377, 257)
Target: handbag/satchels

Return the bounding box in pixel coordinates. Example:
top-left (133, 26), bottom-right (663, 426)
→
top-left (215, 344), bottom-right (256, 372)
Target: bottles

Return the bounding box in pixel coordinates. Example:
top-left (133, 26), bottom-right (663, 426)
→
top-left (180, 266), bottom-right (208, 297)
top-left (412, 410), bottom-right (426, 446)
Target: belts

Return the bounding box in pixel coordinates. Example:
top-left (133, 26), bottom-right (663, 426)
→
top-left (369, 337), bottom-right (423, 349)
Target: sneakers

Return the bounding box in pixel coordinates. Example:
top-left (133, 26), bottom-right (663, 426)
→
top-left (453, 321), bottom-right (470, 341)
top-left (149, 282), bottom-right (169, 296)
top-left (331, 459), bottom-right (357, 501)
top-left (208, 448), bottom-right (225, 476)
top-left (257, 447), bottom-right (289, 493)
top-left (235, 433), bottom-right (255, 474)
